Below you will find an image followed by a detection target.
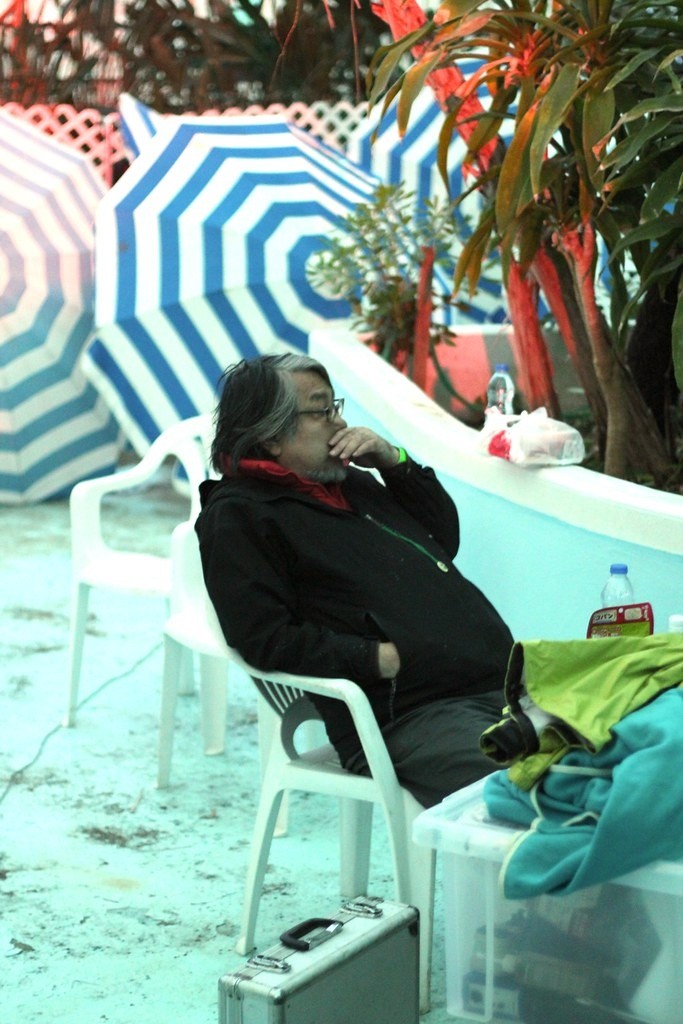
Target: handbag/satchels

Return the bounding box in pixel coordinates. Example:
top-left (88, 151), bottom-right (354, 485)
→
top-left (467, 405), bottom-right (585, 466)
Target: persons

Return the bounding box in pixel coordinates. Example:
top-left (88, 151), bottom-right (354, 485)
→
top-left (191, 352), bottom-right (543, 814)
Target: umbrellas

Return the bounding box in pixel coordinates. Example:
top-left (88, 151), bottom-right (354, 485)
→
top-left (0, 36), bottom-right (681, 509)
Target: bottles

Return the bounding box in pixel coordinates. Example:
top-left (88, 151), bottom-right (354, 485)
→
top-left (484, 363), bottom-right (514, 425)
top-left (602, 564), bottom-right (635, 609)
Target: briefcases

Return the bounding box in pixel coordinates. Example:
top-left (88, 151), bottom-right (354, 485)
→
top-left (218, 894), bottom-right (421, 1024)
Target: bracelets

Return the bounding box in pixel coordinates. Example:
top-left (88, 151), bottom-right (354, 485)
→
top-left (393, 445), bottom-right (407, 465)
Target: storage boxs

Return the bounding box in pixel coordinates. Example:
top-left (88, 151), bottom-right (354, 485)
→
top-left (413, 770), bottom-right (683, 1024)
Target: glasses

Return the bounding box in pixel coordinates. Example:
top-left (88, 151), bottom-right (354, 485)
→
top-left (299, 397), bottom-right (345, 423)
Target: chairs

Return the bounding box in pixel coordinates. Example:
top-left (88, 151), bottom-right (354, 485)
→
top-left (64, 415), bottom-right (304, 833)
top-left (226, 637), bottom-right (452, 1020)
